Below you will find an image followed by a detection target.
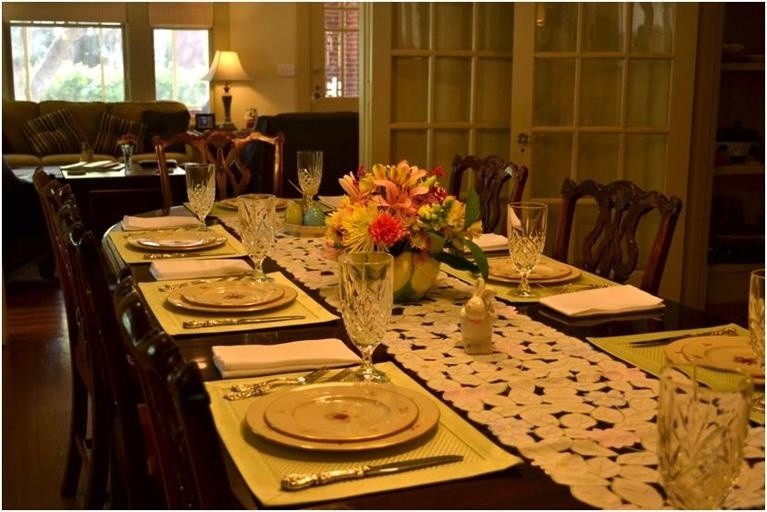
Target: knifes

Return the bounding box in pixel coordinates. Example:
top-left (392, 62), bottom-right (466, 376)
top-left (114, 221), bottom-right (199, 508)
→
top-left (183, 313), bottom-right (308, 330)
top-left (143, 250), bottom-right (226, 259)
top-left (271, 452), bottom-right (464, 495)
top-left (623, 325), bottom-right (741, 348)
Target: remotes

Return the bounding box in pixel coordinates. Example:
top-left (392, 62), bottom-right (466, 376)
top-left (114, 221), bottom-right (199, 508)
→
top-left (138, 159), bottom-right (177, 167)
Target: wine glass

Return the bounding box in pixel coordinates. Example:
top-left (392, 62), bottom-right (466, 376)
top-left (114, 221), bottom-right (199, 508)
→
top-left (237, 194), bottom-right (277, 282)
top-left (185, 163), bottom-right (217, 233)
top-left (744, 266), bottom-right (767, 373)
top-left (294, 147), bottom-right (326, 215)
top-left (336, 252), bottom-right (397, 384)
top-left (505, 201), bottom-right (546, 299)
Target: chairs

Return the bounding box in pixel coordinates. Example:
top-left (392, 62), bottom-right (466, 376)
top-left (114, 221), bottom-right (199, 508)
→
top-left (194, 111), bottom-right (358, 200)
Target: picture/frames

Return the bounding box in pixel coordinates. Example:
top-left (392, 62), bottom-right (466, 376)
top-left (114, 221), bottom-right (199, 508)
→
top-left (196, 114), bottom-right (215, 130)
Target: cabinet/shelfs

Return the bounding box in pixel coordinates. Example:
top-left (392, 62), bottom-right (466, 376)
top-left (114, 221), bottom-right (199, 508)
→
top-left (708, 61), bottom-right (765, 304)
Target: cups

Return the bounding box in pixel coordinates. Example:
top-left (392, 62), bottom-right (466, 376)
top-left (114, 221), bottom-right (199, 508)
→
top-left (658, 340), bottom-right (751, 508)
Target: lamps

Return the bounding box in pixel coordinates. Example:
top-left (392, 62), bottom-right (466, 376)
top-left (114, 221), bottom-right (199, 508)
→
top-left (210, 51), bottom-right (255, 129)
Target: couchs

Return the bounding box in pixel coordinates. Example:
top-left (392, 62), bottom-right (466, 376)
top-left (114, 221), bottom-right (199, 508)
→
top-left (3, 100), bottom-right (190, 196)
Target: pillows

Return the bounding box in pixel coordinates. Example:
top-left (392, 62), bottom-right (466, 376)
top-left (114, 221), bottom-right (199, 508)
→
top-left (93, 111), bottom-right (153, 156)
top-left (16, 107), bottom-right (90, 158)
top-left (142, 110), bottom-right (190, 154)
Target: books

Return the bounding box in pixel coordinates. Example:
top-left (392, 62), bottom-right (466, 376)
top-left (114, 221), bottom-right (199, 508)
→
top-left (136, 159), bottom-right (177, 169)
top-left (61, 160), bottom-right (128, 176)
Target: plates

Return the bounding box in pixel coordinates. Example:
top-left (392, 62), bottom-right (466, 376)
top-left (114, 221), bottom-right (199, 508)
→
top-left (482, 256), bottom-right (581, 285)
top-left (282, 223), bottom-right (327, 236)
top-left (665, 333), bottom-right (767, 387)
top-left (242, 380), bottom-right (440, 454)
top-left (220, 198), bottom-right (290, 212)
top-left (165, 279), bottom-right (300, 313)
top-left (127, 227), bottom-right (229, 253)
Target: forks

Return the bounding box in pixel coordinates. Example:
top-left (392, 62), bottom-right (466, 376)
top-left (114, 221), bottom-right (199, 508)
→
top-left (225, 363), bottom-right (353, 400)
